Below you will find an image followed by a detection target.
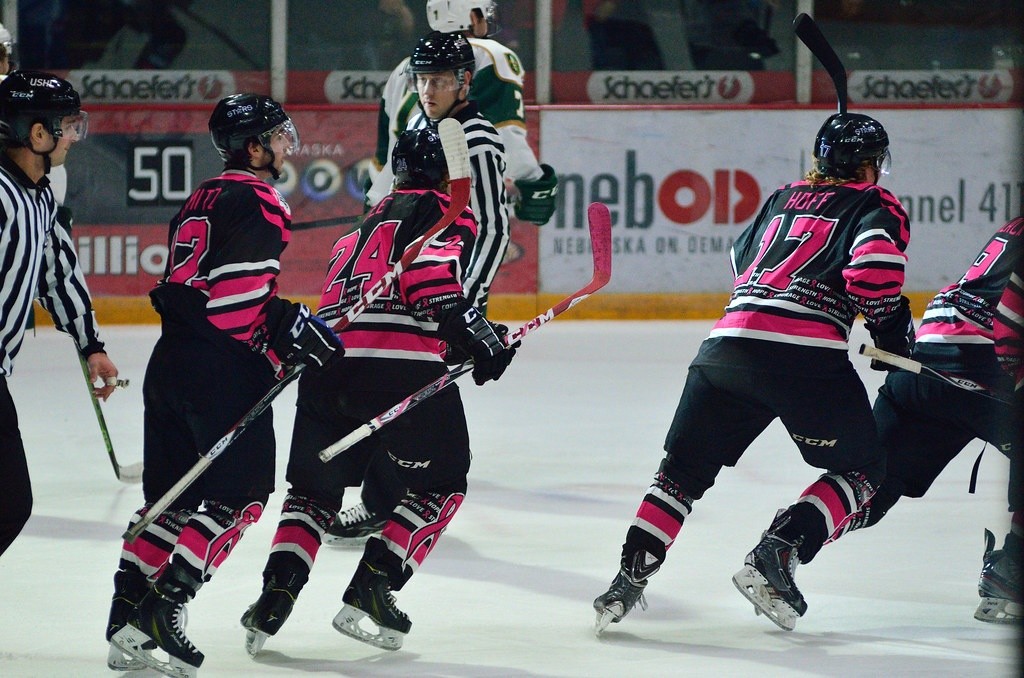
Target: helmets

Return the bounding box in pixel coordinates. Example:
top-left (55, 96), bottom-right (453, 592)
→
top-left (209, 91), bottom-right (301, 171)
top-left (0, 21), bottom-right (14, 56)
top-left (0, 69), bottom-right (90, 156)
top-left (426, 1), bottom-right (508, 39)
top-left (813, 112), bottom-right (892, 178)
top-left (392, 127), bottom-right (448, 186)
top-left (403, 31), bottom-right (476, 90)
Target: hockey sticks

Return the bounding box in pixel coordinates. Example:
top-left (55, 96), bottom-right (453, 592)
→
top-left (121, 118), bottom-right (471, 545)
top-left (77, 348), bottom-right (144, 485)
top-left (318, 202), bottom-right (612, 464)
top-left (858, 343), bottom-right (1024, 409)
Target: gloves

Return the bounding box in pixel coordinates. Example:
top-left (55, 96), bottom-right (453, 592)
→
top-left (863, 296), bottom-right (916, 372)
top-left (513, 164), bottom-right (560, 227)
top-left (271, 301), bottom-right (345, 376)
top-left (435, 301), bottom-right (522, 388)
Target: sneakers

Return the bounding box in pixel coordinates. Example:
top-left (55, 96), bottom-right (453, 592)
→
top-left (241, 571), bottom-right (300, 658)
top-left (106, 571), bottom-right (157, 670)
top-left (332, 536), bottom-right (413, 650)
top-left (322, 500), bottom-right (390, 548)
top-left (111, 586), bottom-right (205, 678)
top-left (733, 507), bottom-right (808, 632)
top-left (592, 538), bottom-right (666, 636)
top-left (974, 527), bottom-right (1024, 623)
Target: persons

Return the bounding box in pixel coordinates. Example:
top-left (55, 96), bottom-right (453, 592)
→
top-left (583, 0), bottom-right (781, 69)
top-left (365, 0), bottom-right (557, 225)
top-left (0, 71), bottom-right (117, 558)
top-left (105, 92), bottom-right (346, 678)
top-left (370, 0), bottom-right (415, 68)
top-left (50, 0), bottom-right (187, 70)
top-left (242, 130), bottom-right (521, 658)
top-left (593, 112), bottom-right (914, 638)
top-left (322, 31), bottom-right (513, 546)
top-left (824, 215), bottom-right (1024, 620)
